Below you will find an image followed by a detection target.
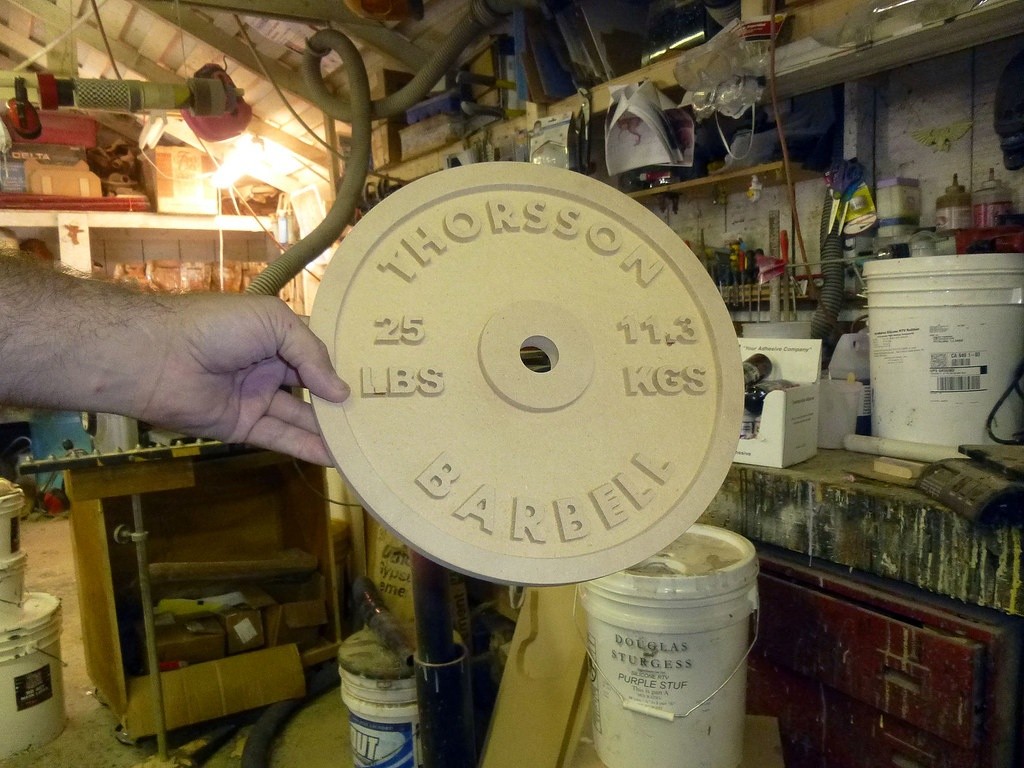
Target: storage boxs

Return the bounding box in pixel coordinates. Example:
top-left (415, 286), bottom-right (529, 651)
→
top-left (0, 108), bottom-right (103, 196)
top-left (731, 337), bottom-right (822, 468)
top-left (124, 547), bottom-right (328, 677)
top-left (139, 147), bottom-right (222, 217)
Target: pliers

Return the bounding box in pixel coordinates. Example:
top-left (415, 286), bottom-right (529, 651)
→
top-left (572, 87), bottom-right (592, 176)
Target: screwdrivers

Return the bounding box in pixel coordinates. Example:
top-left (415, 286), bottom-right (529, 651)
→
top-left (714, 239), bottom-right (765, 324)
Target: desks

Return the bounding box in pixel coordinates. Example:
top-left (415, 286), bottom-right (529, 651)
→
top-left (744, 546), bottom-right (1024, 768)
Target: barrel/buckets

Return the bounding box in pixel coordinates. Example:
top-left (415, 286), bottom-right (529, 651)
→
top-left (861, 253), bottom-right (1024, 448)
top-left (0, 592), bottom-right (66, 758)
top-left (572, 523), bottom-right (760, 768)
top-left (339, 665), bottom-right (423, 768)
top-left (0, 489), bottom-right (28, 630)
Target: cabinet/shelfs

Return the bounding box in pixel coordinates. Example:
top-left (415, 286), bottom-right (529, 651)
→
top-left (360, 0), bottom-right (1024, 768)
top-left (0, 211), bottom-right (275, 420)
top-left (67, 442), bottom-right (341, 739)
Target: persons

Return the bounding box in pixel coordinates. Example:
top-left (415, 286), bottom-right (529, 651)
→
top-left (0, 251), bottom-right (350, 467)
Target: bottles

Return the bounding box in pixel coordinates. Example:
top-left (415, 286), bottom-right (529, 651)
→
top-left (931, 167), bottom-right (972, 235)
top-left (970, 169), bottom-right (1014, 232)
